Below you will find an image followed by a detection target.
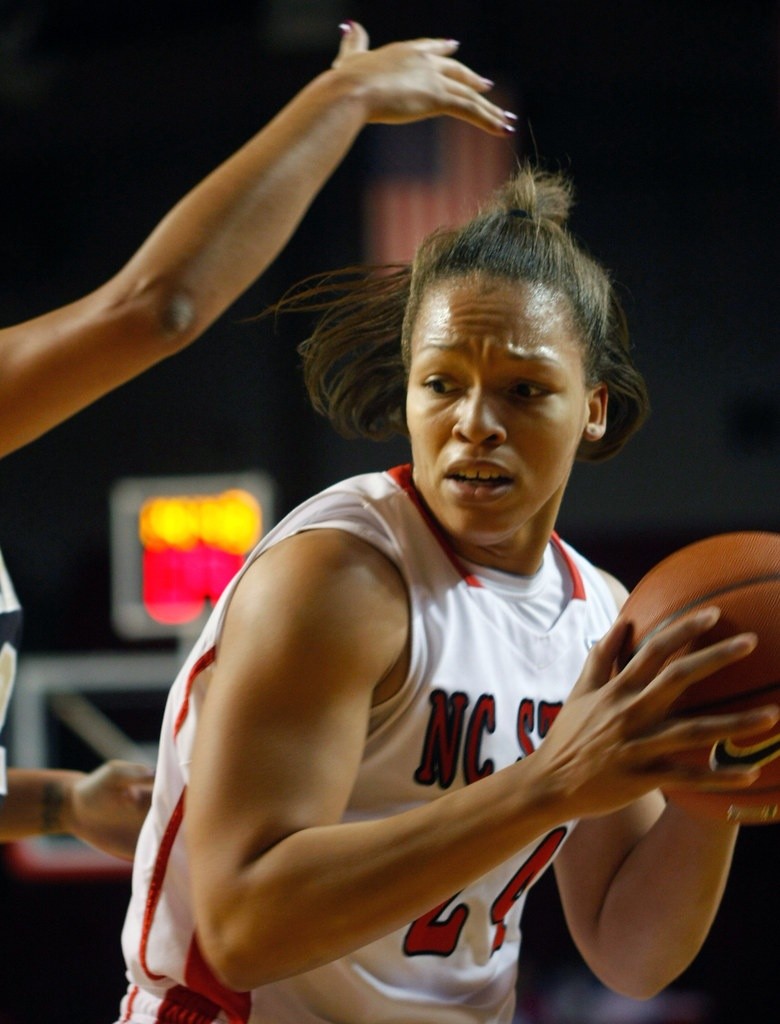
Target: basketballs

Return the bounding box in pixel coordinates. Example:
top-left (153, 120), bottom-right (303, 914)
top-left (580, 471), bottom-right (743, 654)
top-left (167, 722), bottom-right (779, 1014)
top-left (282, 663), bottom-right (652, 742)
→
top-left (607, 530), bottom-right (780, 826)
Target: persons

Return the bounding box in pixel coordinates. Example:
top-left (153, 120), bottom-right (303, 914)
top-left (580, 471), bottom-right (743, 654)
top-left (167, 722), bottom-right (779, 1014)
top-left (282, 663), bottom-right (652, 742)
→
top-left (117, 162), bottom-right (780, 1024)
top-left (0, 18), bottom-right (517, 863)
